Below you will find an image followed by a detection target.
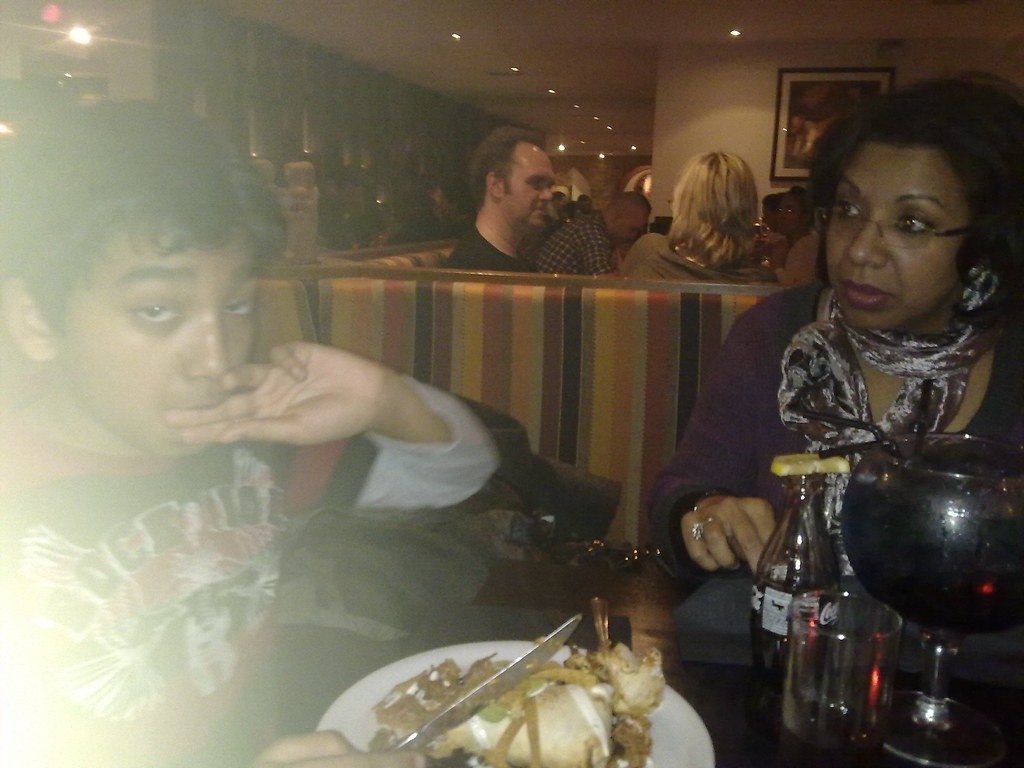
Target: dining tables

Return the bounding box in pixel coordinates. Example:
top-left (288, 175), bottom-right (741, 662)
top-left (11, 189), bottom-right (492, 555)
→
top-left (230, 529), bottom-right (1022, 768)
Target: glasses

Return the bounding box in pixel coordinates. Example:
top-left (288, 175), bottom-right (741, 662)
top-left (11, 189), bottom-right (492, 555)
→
top-left (776, 208), bottom-right (793, 216)
top-left (813, 205), bottom-right (973, 250)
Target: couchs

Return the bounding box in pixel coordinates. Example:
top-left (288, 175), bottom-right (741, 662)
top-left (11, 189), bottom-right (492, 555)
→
top-left (246, 235), bottom-right (795, 524)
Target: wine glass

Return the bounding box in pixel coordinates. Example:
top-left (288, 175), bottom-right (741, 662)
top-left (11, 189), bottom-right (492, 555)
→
top-left (841, 431), bottom-right (1023, 767)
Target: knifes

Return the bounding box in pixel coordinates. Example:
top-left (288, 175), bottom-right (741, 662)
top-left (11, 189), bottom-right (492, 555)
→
top-left (382, 615), bottom-right (580, 751)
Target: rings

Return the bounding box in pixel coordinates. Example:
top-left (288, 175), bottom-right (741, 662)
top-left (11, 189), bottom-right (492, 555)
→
top-left (693, 516), bottom-right (723, 544)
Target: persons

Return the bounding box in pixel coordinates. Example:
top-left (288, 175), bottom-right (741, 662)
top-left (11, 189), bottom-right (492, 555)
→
top-left (771, 186), bottom-right (821, 287)
top-left (642, 70), bottom-right (1024, 578)
top-left (620, 150), bottom-right (778, 285)
top-left (553, 191), bottom-right (592, 222)
top-left (443, 124), bottom-right (555, 273)
top-left (1, 101), bottom-right (531, 768)
top-left (535, 193), bottom-right (652, 275)
top-left (753, 194), bottom-right (787, 277)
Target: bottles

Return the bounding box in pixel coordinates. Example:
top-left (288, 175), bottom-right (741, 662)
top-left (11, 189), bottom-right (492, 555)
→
top-left (749, 473), bottom-right (839, 672)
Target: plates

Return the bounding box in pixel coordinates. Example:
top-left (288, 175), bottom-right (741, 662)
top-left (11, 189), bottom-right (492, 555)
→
top-left (316, 643), bottom-right (716, 768)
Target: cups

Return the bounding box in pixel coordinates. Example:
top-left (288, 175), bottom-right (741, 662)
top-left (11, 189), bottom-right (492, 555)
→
top-left (779, 591), bottom-right (902, 751)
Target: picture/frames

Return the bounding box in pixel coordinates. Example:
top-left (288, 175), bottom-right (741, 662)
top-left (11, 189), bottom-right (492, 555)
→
top-left (769, 66), bottom-right (894, 182)
